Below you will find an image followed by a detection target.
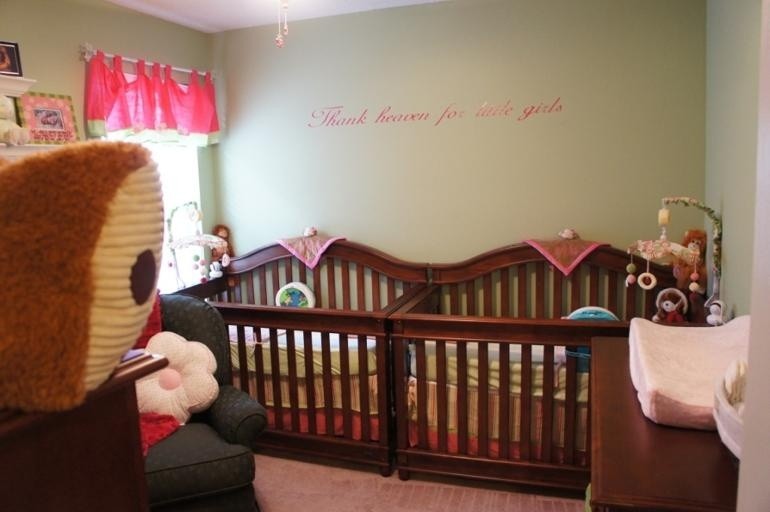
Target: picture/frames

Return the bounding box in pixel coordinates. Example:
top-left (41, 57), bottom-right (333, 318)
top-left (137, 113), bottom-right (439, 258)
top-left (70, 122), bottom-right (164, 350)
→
top-left (0, 39), bottom-right (82, 146)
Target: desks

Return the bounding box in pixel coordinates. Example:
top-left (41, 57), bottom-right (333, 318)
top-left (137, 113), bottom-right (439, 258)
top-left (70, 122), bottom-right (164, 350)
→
top-left (590, 336), bottom-right (740, 512)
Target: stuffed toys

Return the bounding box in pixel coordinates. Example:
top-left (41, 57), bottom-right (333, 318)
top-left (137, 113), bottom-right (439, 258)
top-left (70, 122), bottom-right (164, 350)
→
top-left (0, 93), bottom-right (31, 146)
top-left (1, 143), bottom-right (166, 412)
top-left (651, 288), bottom-right (688, 322)
top-left (673, 230), bottom-right (708, 295)
top-left (211, 224), bottom-right (235, 261)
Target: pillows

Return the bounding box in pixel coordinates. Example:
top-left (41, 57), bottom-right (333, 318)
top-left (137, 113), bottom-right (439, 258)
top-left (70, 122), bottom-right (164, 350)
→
top-left (123, 323), bottom-right (220, 425)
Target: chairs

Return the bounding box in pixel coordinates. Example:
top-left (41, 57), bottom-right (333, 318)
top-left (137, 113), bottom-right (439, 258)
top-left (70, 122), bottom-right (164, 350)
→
top-left (138, 291), bottom-right (269, 506)
